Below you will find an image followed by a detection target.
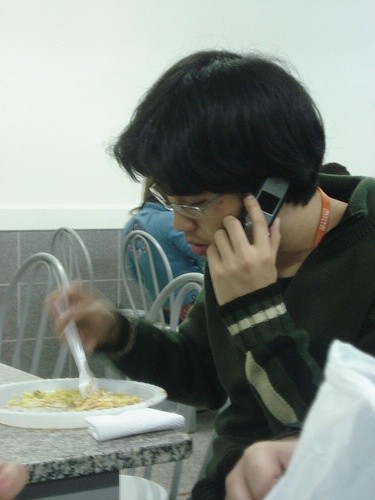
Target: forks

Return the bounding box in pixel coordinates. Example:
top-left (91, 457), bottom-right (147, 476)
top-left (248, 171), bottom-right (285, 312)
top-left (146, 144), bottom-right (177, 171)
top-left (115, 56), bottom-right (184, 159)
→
top-left (56, 294), bottom-right (99, 398)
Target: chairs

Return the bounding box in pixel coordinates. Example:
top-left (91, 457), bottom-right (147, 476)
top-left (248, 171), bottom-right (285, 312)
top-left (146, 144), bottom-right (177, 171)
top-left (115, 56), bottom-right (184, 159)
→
top-left (0, 228), bottom-right (231, 500)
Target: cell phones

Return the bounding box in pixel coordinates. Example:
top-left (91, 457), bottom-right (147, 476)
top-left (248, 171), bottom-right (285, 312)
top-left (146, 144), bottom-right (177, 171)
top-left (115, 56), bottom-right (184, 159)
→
top-left (241, 175), bottom-right (290, 245)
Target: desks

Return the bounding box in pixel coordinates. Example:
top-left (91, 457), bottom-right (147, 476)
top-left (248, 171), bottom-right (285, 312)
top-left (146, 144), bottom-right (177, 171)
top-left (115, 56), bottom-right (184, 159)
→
top-left (0, 359), bottom-right (193, 500)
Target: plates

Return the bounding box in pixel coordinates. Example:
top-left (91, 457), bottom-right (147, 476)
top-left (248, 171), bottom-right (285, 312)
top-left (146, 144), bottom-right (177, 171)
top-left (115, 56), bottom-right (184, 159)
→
top-left (0, 378), bottom-right (167, 430)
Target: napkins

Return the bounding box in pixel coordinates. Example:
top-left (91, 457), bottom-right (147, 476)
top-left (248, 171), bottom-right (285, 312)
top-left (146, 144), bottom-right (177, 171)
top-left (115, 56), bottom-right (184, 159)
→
top-left (84, 408), bottom-right (185, 441)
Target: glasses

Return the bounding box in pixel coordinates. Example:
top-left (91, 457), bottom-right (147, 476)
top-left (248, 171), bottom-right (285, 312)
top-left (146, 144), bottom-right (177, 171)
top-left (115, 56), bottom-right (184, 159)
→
top-left (148, 182), bottom-right (223, 219)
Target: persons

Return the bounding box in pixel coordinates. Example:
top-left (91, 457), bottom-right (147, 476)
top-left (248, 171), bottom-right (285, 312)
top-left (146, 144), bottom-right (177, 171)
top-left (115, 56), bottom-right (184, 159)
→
top-left (43, 50), bottom-right (375, 500)
top-left (124, 177), bottom-right (206, 324)
